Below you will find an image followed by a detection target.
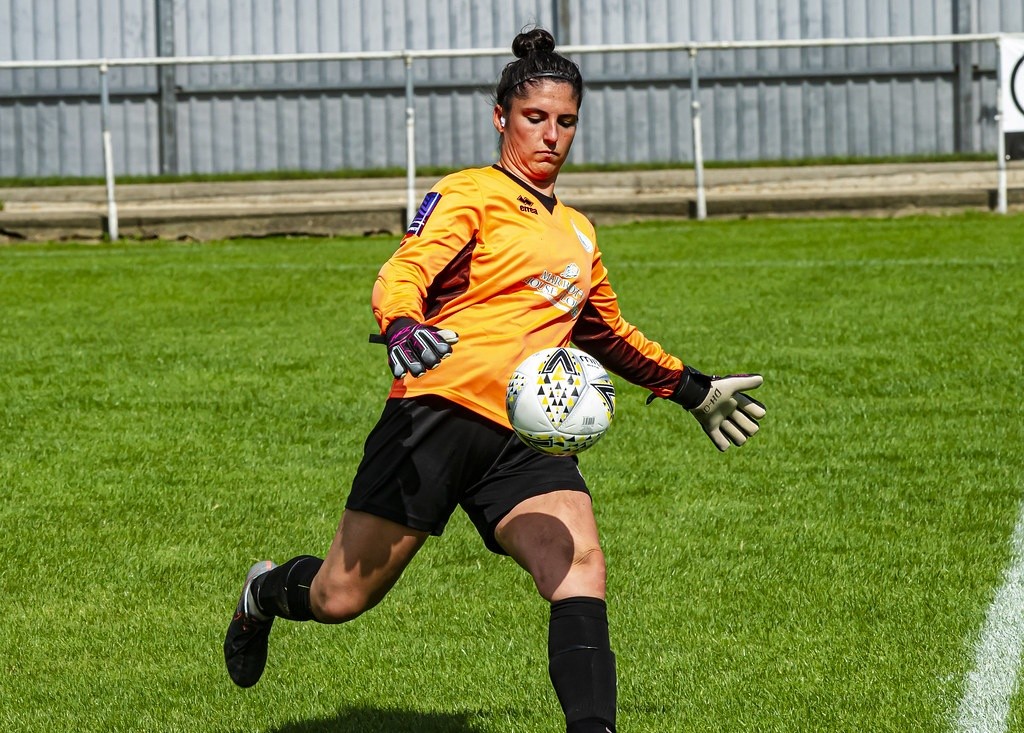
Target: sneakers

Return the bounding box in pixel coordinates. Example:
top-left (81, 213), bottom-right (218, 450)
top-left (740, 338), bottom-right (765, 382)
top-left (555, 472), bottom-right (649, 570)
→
top-left (224, 556), bottom-right (280, 690)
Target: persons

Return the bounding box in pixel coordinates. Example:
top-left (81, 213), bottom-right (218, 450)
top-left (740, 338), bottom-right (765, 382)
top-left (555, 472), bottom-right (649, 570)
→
top-left (226, 31), bottom-right (770, 733)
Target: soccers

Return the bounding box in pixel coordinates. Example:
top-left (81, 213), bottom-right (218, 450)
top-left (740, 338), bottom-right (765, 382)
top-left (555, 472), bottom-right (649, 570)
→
top-left (504, 347), bottom-right (618, 457)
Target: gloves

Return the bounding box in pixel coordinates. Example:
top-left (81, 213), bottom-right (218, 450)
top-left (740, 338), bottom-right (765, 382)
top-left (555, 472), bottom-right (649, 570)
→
top-left (380, 315), bottom-right (459, 389)
top-left (666, 362), bottom-right (770, 454)
